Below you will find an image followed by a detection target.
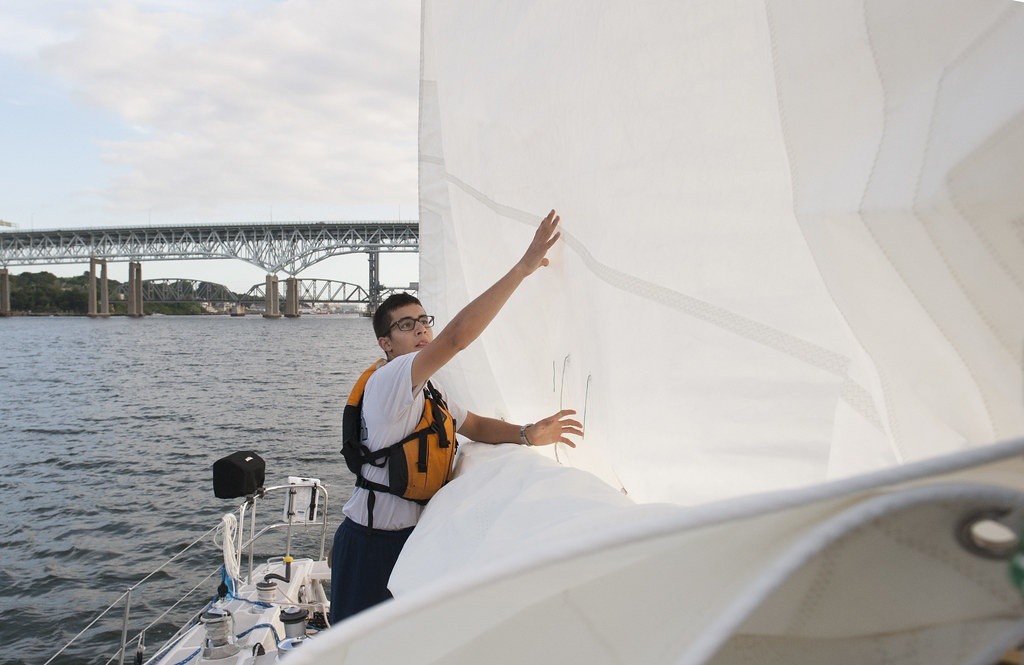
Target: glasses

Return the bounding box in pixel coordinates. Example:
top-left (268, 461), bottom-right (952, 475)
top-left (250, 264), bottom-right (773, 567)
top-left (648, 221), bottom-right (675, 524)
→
top-left (381, 315), bottom-right (434, 337)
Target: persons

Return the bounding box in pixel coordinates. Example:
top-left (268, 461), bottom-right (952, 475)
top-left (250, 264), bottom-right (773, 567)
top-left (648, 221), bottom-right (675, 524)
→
top-left (328, 210), bottom-right (583, 626)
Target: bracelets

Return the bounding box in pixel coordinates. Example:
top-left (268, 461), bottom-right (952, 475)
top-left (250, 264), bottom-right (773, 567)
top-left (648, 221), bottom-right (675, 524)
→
top-left (520, 423), bottom-right (532, 446)
